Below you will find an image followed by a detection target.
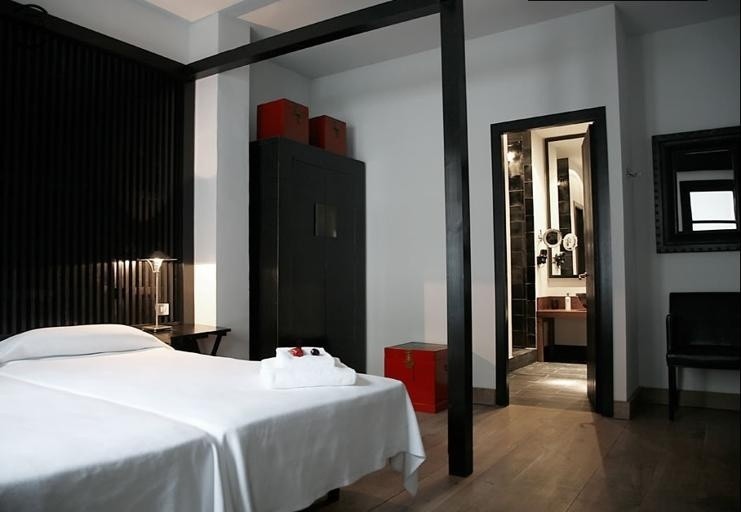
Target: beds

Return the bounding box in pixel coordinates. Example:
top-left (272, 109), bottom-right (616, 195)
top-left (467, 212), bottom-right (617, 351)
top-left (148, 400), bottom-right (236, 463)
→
top-left (1, 373), bottom-right (233, 512)
top-left (1, 347), bottom-right (428, 512)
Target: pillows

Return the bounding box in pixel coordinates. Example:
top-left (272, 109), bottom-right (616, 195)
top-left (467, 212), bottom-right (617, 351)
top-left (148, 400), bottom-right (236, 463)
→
top-left (0, 324), bottom-right (176, 365)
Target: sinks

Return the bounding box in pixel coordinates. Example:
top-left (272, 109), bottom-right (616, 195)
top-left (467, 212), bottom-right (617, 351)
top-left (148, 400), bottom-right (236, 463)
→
top-left (576, 292), bottom-right (586, 308)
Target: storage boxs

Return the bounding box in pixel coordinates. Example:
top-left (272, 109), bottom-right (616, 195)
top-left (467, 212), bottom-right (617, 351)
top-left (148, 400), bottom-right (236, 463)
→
top-left (383, 341), bottom-right (449, 414)
top-left (309, 114), bottom-right (347, 155)
top-left (256, 98), bottom-right (309, 146)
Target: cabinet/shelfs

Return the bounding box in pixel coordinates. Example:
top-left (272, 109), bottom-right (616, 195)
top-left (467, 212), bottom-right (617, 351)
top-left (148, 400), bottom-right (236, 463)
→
top-left (248, 136), bottom-right (367, 373)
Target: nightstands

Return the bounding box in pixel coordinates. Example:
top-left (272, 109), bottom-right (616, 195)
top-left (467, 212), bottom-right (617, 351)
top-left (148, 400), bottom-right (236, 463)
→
top-left (132, 323), bottom-right (232, 356)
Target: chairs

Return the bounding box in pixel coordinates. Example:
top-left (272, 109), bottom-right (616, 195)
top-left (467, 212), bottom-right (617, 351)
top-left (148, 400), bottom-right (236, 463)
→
top-left (665, 292), bottom-right (740, 422)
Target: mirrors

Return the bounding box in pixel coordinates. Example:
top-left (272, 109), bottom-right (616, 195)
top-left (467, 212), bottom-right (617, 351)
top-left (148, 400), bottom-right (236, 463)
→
top-left (652, 126), bottom-right (741, 253)
top-left (545, 133), bottom-right (586, 279)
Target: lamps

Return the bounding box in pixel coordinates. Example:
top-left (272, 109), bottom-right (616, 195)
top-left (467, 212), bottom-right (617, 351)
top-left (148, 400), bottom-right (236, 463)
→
top-left (137, 250), bottom-right (179, 334)
